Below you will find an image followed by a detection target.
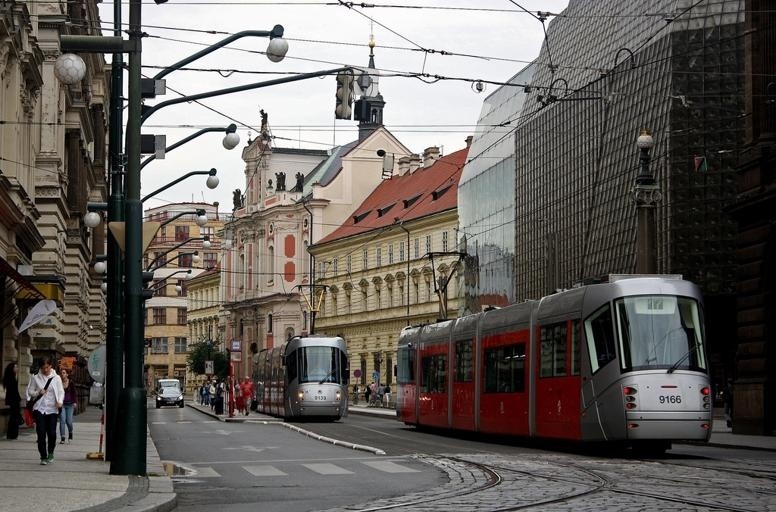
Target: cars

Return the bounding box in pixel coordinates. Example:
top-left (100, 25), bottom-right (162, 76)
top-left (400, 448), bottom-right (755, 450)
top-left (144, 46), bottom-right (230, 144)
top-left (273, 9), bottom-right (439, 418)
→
top-left (154, 386), bottom-right (185, 409)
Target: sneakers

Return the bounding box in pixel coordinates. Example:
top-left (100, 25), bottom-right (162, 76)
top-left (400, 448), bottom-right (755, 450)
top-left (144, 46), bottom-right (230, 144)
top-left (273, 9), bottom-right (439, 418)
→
top-left (41, 432), bottom-right (72, 465)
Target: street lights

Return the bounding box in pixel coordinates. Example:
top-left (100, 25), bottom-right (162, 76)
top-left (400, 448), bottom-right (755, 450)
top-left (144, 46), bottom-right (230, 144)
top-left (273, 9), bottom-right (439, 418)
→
top-left (632, 132), bottom-right (662, 274)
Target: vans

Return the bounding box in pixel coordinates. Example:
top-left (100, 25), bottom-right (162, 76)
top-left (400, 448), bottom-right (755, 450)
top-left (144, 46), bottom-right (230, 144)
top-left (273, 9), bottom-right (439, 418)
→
top-left (155, 379), bottom-right (183, 394)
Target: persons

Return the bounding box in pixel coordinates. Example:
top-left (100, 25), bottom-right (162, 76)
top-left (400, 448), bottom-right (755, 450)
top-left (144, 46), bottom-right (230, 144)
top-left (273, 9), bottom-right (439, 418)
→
top-left (259, 108), bottom-right (268, 133)
top-left (295, 171), bottom-right (305, 193)
top-left (26, 355), bottom-right (68, 466)
top-left (197, 374), bottom-right (260, 417)
top-left (232, 188), bottom-right (241, 210)
top-left (351, 379), bottom-right (394, 409)
top-left (274, 171), bottom-right (286, 192)
top-left (2, 360), bottom-right (22, 441)
top-left (58, 368), bottom-right (78, 445)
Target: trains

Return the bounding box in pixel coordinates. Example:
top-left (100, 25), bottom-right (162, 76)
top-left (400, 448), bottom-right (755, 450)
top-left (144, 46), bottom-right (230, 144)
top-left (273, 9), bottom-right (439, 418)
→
top-left (397, 273), bottom-right (713, 454)
top-left (249, 334), bottom-right (347, 421)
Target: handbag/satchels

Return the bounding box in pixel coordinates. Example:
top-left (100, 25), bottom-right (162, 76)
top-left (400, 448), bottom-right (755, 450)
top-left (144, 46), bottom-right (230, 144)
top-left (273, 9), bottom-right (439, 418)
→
top-left (23, 407), bottom-right (35, 427)
